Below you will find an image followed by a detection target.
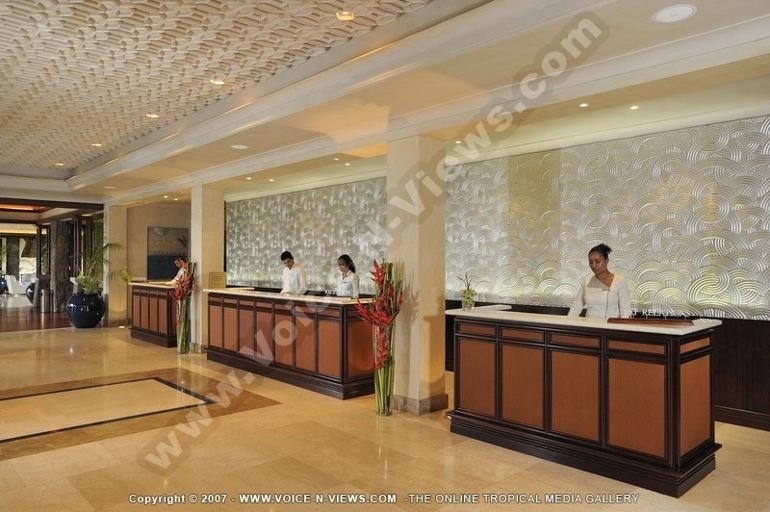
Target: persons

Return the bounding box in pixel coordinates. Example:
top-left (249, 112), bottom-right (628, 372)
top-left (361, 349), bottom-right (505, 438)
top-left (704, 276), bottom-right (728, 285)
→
top-left (567, 242), bottom-right (631, 320)
top-left (280, 251), bottom-right (309, 295)
top-left (165, 255), bottom-right (188, 285)
top-left (335, 254), bottom-right (361, 299)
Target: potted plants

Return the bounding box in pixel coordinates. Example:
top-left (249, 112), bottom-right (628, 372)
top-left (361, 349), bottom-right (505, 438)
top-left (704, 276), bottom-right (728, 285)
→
top-left (457, 275), bottom-right (487, 311)
top-left (64, 216), bottom-right (130, 327)
top-left (26, 235), bottom-right (49, 304)
top-left (353, 257), bottom-right (404, 417)
top-left (169, 256), bottom-right (199, 354)
top-left (0, 240), bottom-right (18, 294)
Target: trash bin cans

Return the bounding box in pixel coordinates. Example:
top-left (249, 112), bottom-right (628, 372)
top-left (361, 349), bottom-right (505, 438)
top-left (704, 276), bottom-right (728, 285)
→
top-left (40, 288), bottom-right (50, 313)
